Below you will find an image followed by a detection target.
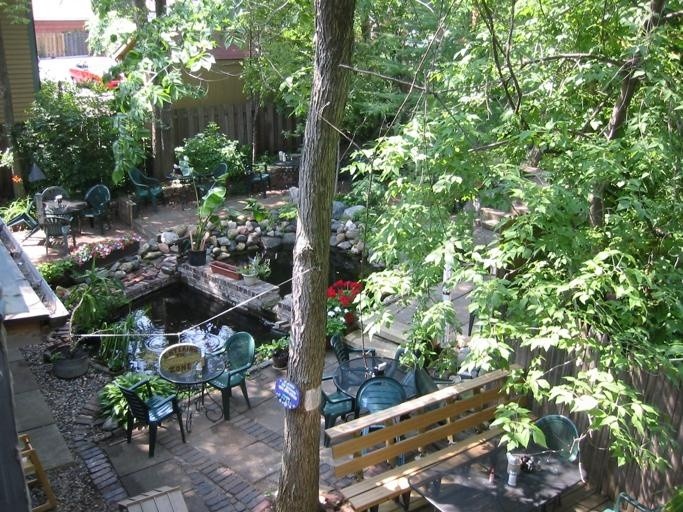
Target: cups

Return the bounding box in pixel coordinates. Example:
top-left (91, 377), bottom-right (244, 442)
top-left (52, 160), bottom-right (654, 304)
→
top-left (507, 457), bottom-right (521, 475)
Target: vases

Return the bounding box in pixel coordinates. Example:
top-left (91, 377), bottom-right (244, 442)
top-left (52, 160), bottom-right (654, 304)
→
top-left (210, 260), bottom-right (242, 281)
top-left (241, 272), bottom-right (257, 286)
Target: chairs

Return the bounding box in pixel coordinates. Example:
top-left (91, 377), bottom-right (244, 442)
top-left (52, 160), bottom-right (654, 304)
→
top-left (127, 166), bottom-right (166, 213)
top-left (529, 414), bottom-right (581, 507)
top-left (201, 331), bottom-right (256, 421)
top-left (319, 336), bottom-right (475, 467)
top-left (245, 163), bottom-right (271, 199)
top-left (196, 163), bottom-right (227, 205)
top-left (41, 184), bottom-right (111, 257)
top-left (7, 200), bottom-right (40, 243)
top-left (119, 379), bottom-right (185, 457)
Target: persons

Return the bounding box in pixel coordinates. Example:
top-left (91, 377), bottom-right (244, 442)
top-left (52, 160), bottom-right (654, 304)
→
top-left (412, 335), bottom-right (441, 369)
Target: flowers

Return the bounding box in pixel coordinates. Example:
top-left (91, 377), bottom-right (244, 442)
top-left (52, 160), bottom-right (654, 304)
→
top-left (69, 231), bottom-right (145, 269)
top-left (324, 279), bottom-right (366, 335)
top-left (233, 251), bottom-right (262, 275)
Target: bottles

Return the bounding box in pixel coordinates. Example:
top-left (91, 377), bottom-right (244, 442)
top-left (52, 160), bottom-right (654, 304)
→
top-left (506, 470), bottom-right (518, 486)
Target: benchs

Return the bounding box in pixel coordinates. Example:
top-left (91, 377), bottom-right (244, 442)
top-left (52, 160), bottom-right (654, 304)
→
top-left (324, 369), bottom-right (539, 512)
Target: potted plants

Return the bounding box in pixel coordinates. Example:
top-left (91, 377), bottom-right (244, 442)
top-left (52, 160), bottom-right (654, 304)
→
top-left (489, 401), bottom-right (550, 487)
top-left (186, 173), bottom-right (229, 266)
top-left (43, 258), bottom-right (153, 379)
top-left (256, 336), bottom-right (289, 368)
